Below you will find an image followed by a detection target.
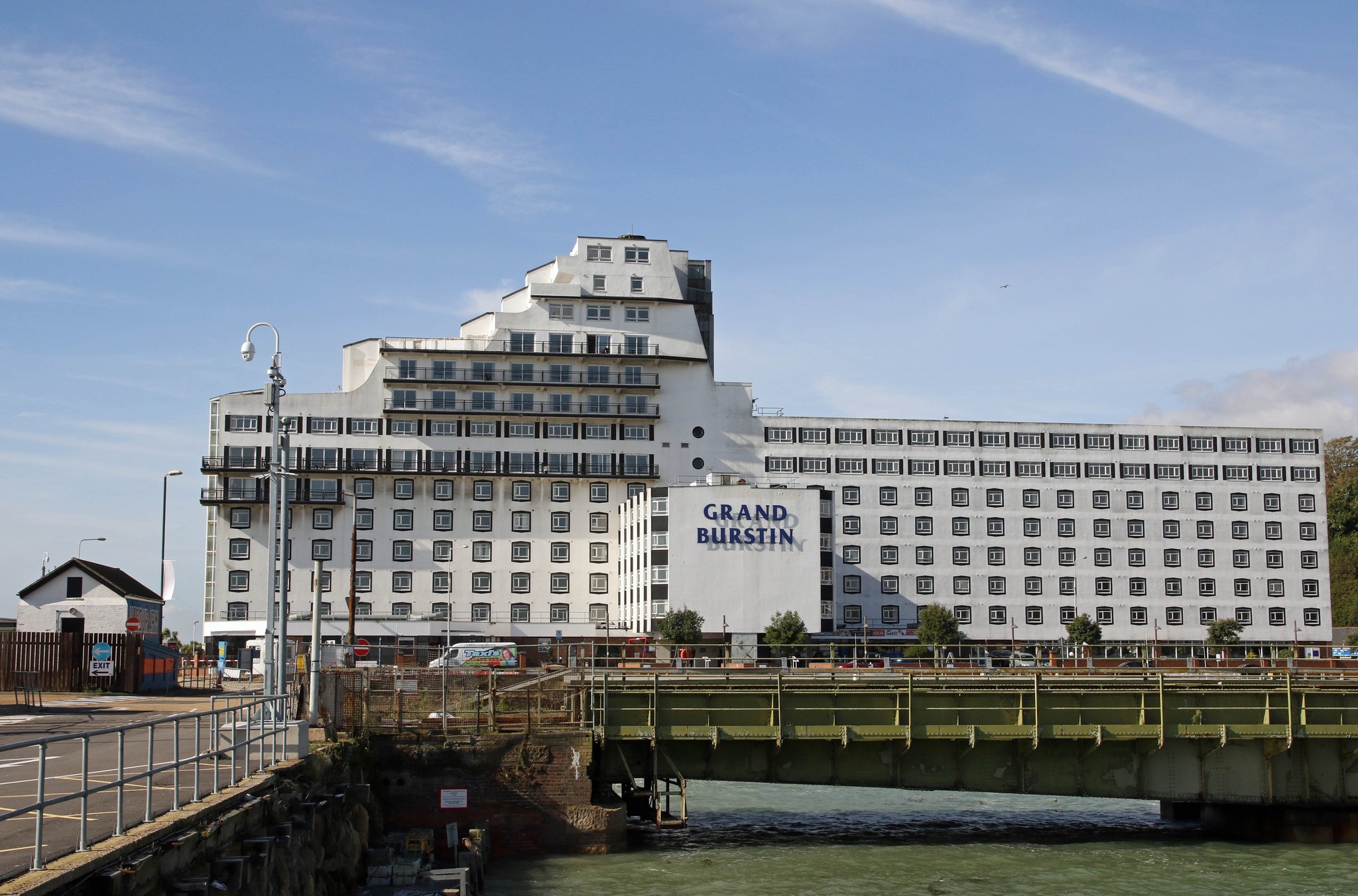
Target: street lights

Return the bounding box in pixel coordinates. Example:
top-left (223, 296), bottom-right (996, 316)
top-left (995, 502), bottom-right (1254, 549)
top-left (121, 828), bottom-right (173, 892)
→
top-left (723, 615), bottom-right (729, 667)
top-left (1154, 618), bottom-right (1161, 666)
top-left (605, 614), bottom-right (615, 667)
top-left (1011, 617), bottom-right (1018, 653)
top-left (241, 321), bottom-right (288, 720)
top-left (159, 470), bottom-right (183, 644)
top-left (448, 545), bottom-right (469, 649)
top-left (863, 615), bottom-right (871, 664)
top-left (78, 536), bottom-right (106, 560)
top-left (193, 621), bottom-right (199, 657)
top-left (1294, 620), bottom-right (1302, 657)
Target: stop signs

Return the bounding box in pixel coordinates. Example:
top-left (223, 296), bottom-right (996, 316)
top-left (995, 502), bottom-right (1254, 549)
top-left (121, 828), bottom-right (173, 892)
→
top-left (354, 639), bottom-right (369, 656)
top-left (126, 617), bottom-right (141, 632)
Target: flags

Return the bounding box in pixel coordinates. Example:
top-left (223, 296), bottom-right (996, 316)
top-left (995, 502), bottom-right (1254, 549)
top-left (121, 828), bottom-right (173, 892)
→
top-left (163, 560), bottom-right (175, 600)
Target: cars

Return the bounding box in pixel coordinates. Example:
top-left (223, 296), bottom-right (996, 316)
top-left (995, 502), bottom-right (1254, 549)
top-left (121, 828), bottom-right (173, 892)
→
top-left (1008, 652), bottom-right (1036, 667)
top-left (1104, 661), bottom-right (1156, 675)
top-left (988, 650), bottom-right (1013, 667)
top-left (835, 659), bottom-right (884, 667)
top-left (867, 652), bottom-right (912, 663)
top-left (811, 650), bottom-right (842, 663)
top-left (1237, 664), bottom-right (1267, 675)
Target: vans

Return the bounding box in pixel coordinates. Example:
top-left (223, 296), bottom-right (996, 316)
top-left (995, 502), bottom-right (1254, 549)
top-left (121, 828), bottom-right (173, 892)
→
top-left (429, 643), bottom-right (517, 672)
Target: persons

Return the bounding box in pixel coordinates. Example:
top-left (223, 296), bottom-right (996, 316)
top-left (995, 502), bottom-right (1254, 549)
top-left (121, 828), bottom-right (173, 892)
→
top-left (502, 647), bottom-right (517, 666)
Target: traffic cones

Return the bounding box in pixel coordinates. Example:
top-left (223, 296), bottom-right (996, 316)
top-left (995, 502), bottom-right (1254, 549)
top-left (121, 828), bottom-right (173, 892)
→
top-left (215, 669), bottom-right (225, 688)
